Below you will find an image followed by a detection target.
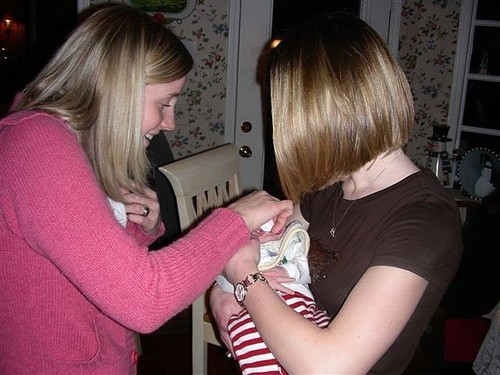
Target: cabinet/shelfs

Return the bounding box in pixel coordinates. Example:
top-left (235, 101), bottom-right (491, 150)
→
top-left (442, 0), bottom-right (500, 202)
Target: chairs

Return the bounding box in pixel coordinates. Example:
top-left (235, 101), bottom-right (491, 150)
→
top-left (157, 143), bottom-right (247, 375)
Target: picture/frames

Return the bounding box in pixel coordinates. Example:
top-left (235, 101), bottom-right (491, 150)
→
top-left (119, 0), bottom-right (196, 20)
top-left (457, 148), bottom-right (500, 202)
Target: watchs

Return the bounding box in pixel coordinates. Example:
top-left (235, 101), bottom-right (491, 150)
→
top-left (232, 273), bottom-right (267, 305)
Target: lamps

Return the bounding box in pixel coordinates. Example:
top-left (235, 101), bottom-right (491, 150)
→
top-left (4, 19), bottom-right (13, 45)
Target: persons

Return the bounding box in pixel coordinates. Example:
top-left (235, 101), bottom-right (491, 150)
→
top-left (0, 4), bottom-right (296, 375)
top-left (204, 13), bottom-right (464, 375)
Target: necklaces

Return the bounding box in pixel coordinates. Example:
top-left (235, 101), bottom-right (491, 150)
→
top-left (329, 147), bottom-right (402, 238)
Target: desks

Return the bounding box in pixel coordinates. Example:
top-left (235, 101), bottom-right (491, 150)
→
top-left (420, 191), bottom-right (500, 375)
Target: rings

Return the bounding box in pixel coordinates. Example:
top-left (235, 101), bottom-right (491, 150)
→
top-left (225, 351), bottom-right (232, 357)
top-left (141, 208), bottom-right (150, 217)
